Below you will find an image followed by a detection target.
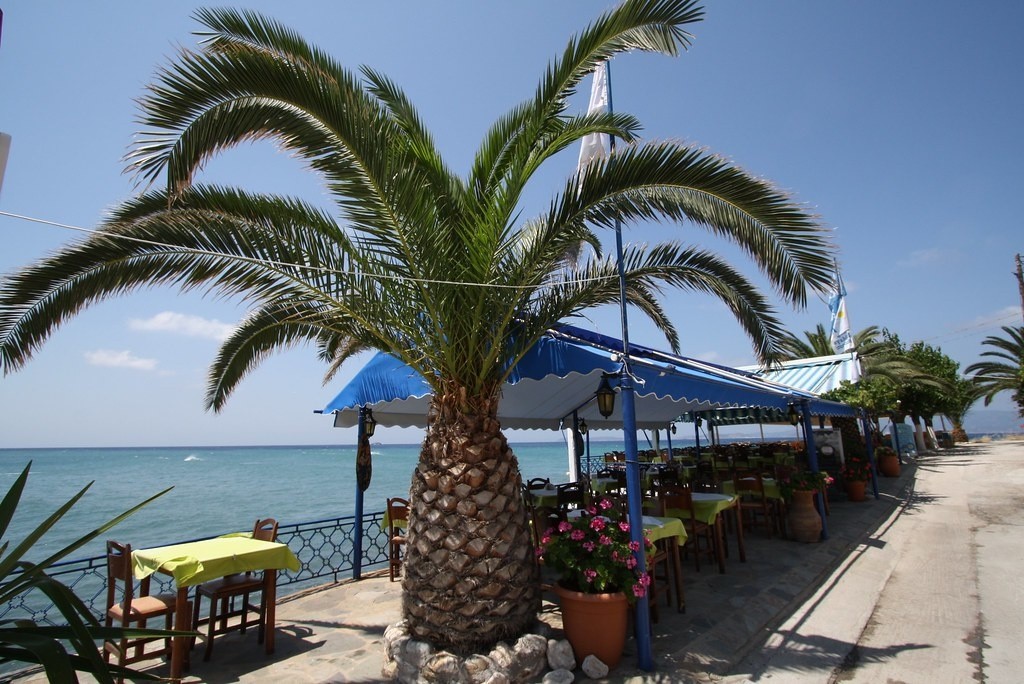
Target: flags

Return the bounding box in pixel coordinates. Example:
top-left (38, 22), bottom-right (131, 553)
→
top-left (828, 261), bottom-right (856, 354)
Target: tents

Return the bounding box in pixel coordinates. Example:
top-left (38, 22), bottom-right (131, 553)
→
top-left (313, 299), bottom-right (879, 674)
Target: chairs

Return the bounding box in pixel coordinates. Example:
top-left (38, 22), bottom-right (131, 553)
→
top-left (190, 518), bottom-right (279, 664)
top-left (102, 540), bottom-right (193, 684)
top-left (521, 442), bottom-right (790, 641)
top-left (387, 497), bottom-right (410, 582)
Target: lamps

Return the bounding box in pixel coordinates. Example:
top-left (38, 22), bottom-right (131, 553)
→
top-left (578, 416), bottom-right (588, 435)
top-left (594, 371), bottom-right (621, 420)
top-left (670, 423), bottom-right (677, 435)
top-left (787, 403), bottom-right (801, 426)
top-left (362, 408), bottom-right (378, 438)
top-left (697, 415), bottom-right (702, 427)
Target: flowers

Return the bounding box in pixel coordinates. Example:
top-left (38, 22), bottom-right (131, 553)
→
top-left (838, 453), bottom-right (873, 485)
top-left (875, 446), bottom-right (896, 456)
top-left (534, 498), bottom-right (657, 604)
top-left (775, 471), bottom-right (835, 495)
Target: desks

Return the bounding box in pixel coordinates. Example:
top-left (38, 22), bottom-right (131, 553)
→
top-left (130, 532), bottom-right (301, 684)
top-left (380, 451), bottom-right (831, 640)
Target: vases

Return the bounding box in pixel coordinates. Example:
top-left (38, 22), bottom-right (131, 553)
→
top-left (552, 575), bottom-right (629, 671)
top-left (847, 479), bottom-right (866, 502)
top-left (787, 489), bottom-right (823, 543)
top-left (879, 456), bottom-right (900, 477)
